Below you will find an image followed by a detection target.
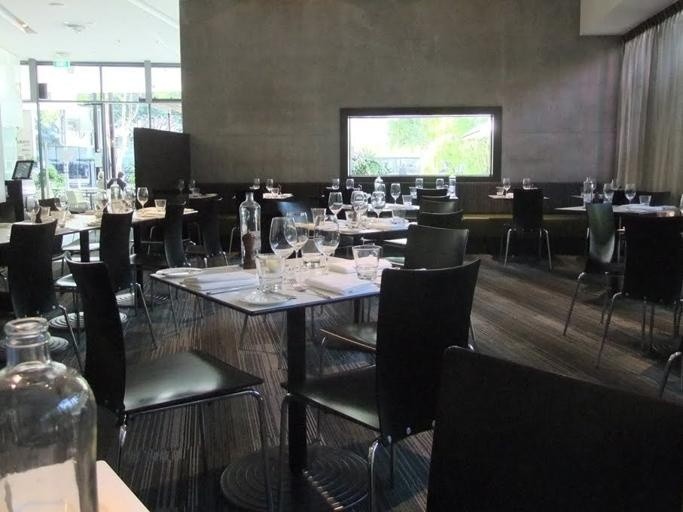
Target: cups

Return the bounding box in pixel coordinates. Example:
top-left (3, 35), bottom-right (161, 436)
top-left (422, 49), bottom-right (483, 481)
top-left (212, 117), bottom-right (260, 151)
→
top-left (678, 193), bottom-right (682, 212)
top-left (350, 244), bottom-right (383, 281)
top-left (638, 196), bottom-right (652, 208)
top-left (254, 254), bottom-right (284, 292)
top-left (495, 186), bottom-right (504, 196)
top-left (300, 236), bottom-right (325, 269)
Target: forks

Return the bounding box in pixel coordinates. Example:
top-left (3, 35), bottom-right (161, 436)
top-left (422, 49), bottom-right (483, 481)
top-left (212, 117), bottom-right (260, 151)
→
top-left (288, 278), bottom-right (331, 301)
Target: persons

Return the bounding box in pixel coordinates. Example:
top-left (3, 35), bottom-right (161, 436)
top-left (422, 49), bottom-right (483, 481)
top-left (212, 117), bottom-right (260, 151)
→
top-left (104, 170), bottom-right (126, 192)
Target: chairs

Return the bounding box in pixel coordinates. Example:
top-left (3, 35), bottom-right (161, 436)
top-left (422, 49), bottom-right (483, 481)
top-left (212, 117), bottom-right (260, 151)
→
top-left (487, 188), bottom-right (681, 401)
top-left (422, 342), bottom-right (681, 512)
top-left (0, 187), bottom-right (482, 512)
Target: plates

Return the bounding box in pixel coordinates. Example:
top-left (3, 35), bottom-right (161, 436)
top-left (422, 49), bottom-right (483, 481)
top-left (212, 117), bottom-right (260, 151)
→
top-left (154, 266), bottom-right (202, 278)
top-left (237, 292), bottom-right (291, 306)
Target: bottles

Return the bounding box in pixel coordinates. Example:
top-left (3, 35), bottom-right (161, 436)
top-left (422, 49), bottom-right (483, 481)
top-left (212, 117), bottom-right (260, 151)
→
top-left (0, 316), bottom-right (99, 508)
top-left (238, 192), bottom-right (262, 269)
top-left (582, 176), bottom-right (593, 210)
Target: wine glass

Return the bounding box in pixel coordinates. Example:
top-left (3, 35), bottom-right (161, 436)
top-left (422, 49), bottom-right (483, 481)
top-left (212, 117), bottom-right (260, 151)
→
top-left (589, 175), bottom-right (635, 208)
top-left (282, 211), bottom-right (311, 261)
top-left (502, 177), bottom-right (531, 193)
top-left (327, 174), bottom-right (422, 228)
top-left (435, 175), bottom-right (455, 196)
top-left (251, 176), bottom-right (282, 198)
top-left (312, 215), bottom-right (341, 274)
top-left (176, 177), bottom-right (199, 197)
top-left (25, 184), bottom-right (166, 229)
top-left (269, 217), bottom-right (297, 261)
